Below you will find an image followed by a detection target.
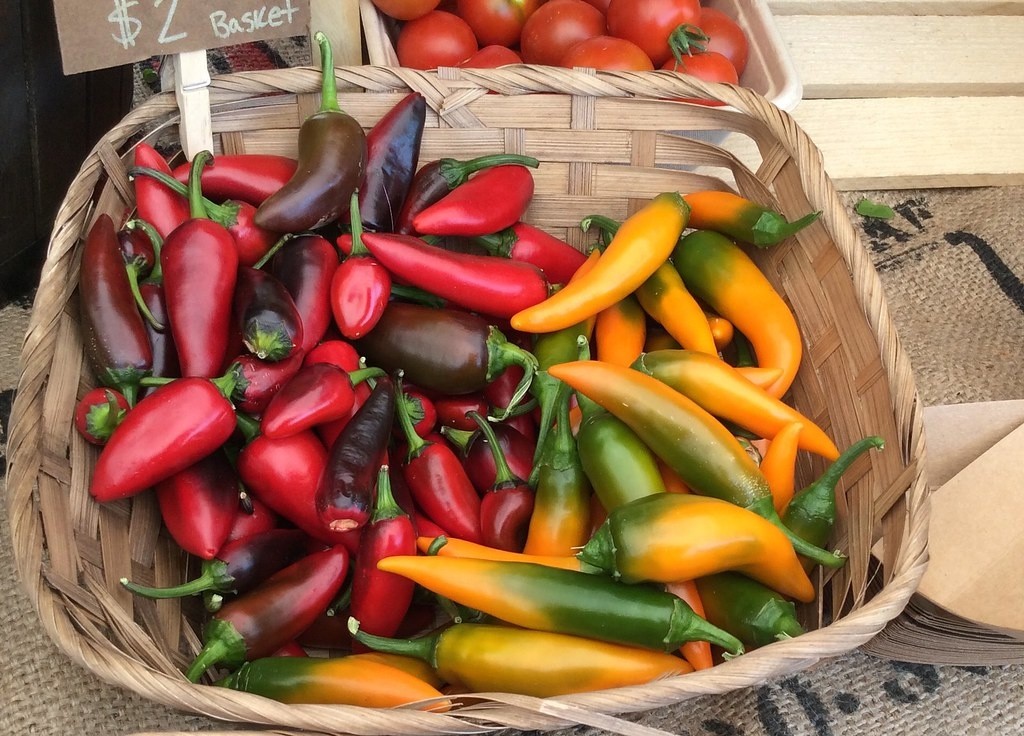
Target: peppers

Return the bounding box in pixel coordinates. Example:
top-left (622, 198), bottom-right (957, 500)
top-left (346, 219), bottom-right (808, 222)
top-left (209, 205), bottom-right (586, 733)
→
top-left (75, 33), bottom-right (886, 711)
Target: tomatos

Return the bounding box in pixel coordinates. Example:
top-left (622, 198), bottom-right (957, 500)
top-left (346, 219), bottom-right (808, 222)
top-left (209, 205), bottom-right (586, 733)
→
top-left (373, 0), bottom-right (749, 106)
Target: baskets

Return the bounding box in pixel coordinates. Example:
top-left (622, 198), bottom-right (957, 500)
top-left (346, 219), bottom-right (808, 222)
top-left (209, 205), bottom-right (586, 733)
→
top-left (6, 65), bottom-right (932, 736)
